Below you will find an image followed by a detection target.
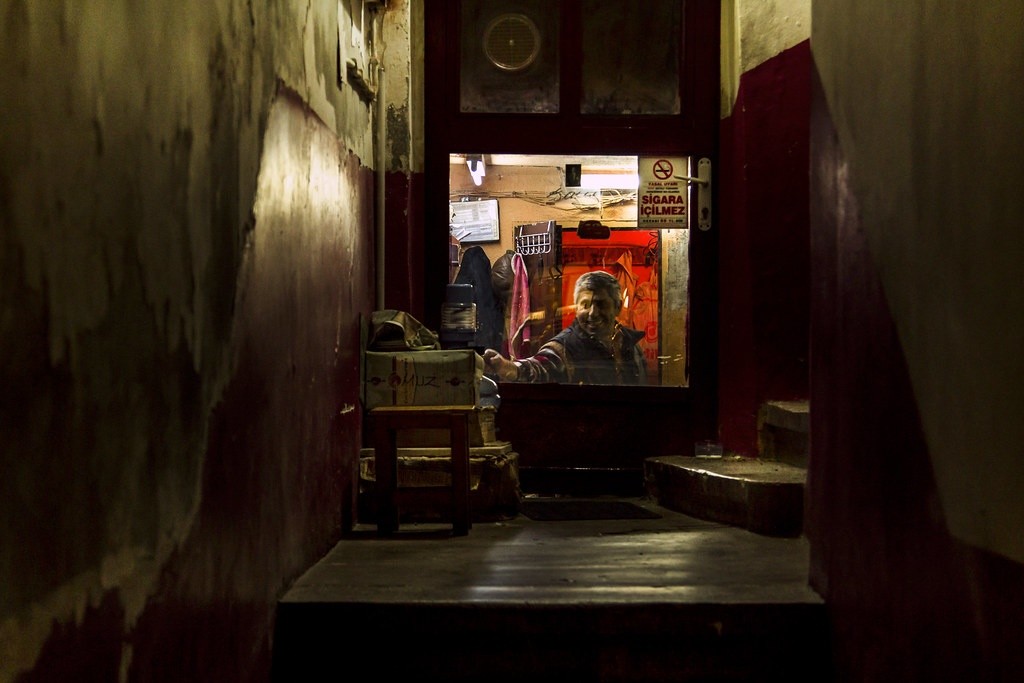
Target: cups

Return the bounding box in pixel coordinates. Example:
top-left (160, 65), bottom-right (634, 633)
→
top-left (694, 439), bottom-right (723, 459)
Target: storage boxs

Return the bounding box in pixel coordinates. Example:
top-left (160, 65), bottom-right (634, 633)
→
top-left (360, 350), bottom-right (519, 524)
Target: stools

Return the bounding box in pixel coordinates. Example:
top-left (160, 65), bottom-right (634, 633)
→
top-left (369, 405), bottom-right (476, 538)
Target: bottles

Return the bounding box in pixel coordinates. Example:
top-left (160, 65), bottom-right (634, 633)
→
top-left (440, 283), bottom-right (477, 348)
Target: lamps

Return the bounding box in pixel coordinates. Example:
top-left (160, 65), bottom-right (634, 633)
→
top-left (466, 154), bottom-right (486, 186)
top-left (439, 283), bottom-right (484, 357)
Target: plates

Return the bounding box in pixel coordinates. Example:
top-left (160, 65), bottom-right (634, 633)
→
top-left (376, 340), bottom-right (406, 349)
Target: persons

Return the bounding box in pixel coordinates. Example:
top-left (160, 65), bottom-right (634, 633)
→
top-left (482, 271), bottom-right (650, 385)
